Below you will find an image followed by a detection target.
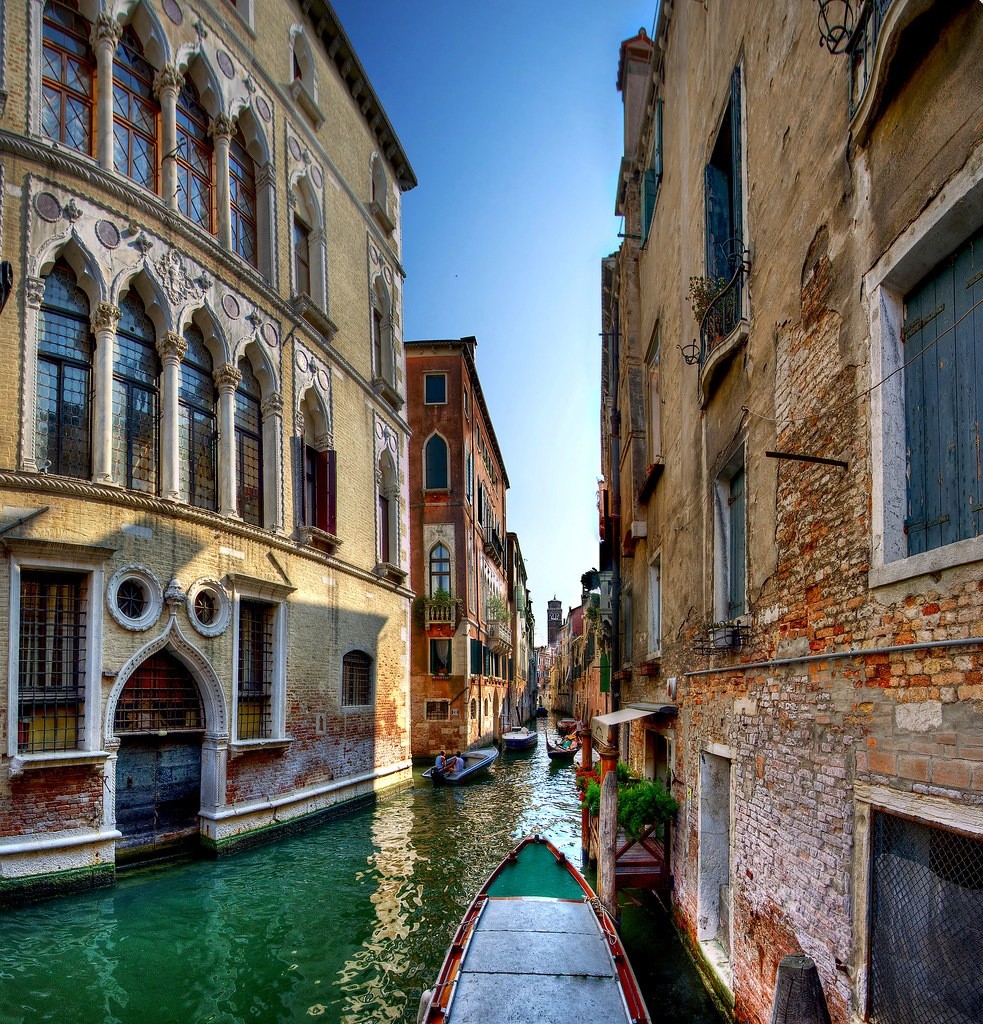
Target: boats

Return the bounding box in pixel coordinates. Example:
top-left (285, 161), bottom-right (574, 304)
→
top-left (502, 728), bottom-right (539, 749)
top-left (546, 733), bottom-right (583, 760)
top-left (422, 745), bottom-right (499, 782)
top-left (556, 718), bottom-right (579, 734)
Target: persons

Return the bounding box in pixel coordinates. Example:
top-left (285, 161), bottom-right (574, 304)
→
top-left (521, 725), bottom-right (529, 734)
top-left (442, 751), bottom-right (464, 772)
top-left (435, 750), bottom-right (446, 769)
top-left (560, 715), bottom-right (586, 750)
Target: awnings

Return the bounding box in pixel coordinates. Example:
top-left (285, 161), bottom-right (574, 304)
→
top-left (591, 702), bottom-right (679, 749)
